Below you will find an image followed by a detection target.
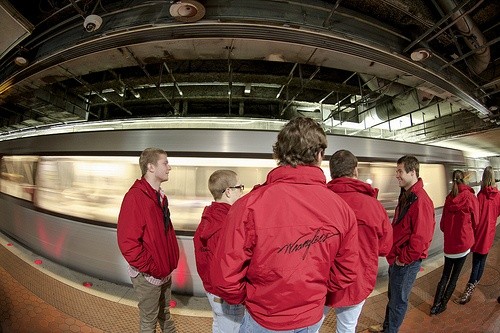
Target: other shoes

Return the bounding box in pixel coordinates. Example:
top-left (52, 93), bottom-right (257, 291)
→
top-left (369, 324), bottom-right (384, 332)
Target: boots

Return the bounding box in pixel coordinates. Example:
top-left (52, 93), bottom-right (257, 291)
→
top-left (460, 283), bottom-right (477, 303)
top-left (430, 282), bottom-right (446, 313)
top-left (435, 285), bottom-right (456, 312)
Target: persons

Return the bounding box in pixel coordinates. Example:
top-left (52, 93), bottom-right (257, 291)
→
top-left (308, 149), bottom-right (393, 333)
top-left (454, 165), bottom-right (500, 305)
top-left (367, 153), bottom-right (435, 333)
top-left (430, 169), bottom-right (479, 315)
top-left (192, 169), bottom-right (247, 333)
top-left (116, 147), bottom-right (179, 333)
top-left (210, 116), bottom-right (361, 333)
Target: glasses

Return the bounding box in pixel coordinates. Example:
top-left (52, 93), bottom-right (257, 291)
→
top-left (222, 184), bottom-right (244, 193)
